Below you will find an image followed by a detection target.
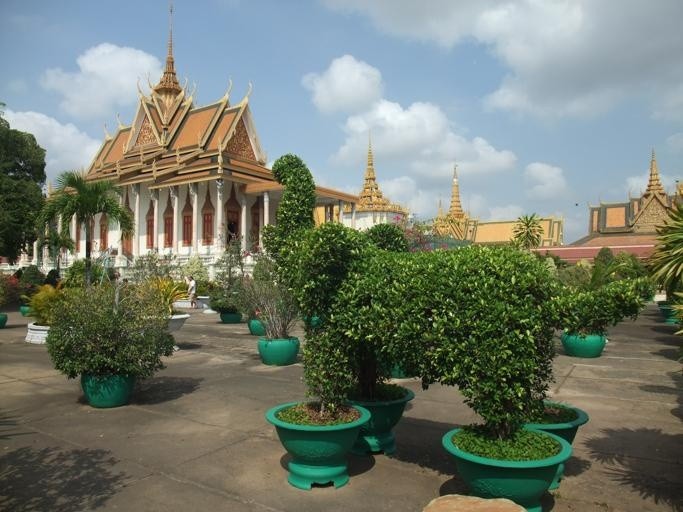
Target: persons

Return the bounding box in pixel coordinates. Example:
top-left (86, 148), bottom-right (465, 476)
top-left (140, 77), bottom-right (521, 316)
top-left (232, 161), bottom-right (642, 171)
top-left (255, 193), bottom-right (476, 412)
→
top-left (187, 276), bottom-right (198, 309)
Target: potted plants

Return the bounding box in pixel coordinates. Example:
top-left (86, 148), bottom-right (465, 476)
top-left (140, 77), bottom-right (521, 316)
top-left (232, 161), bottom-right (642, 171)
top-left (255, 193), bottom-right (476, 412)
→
top-left (1, 273), bottom-right (19, 330)
top-left (192, 222), bottom-right (279, 336)
top-left (247, 154), bottom-right (683, 512)
top-left (19, 239), bottom-right (191, 409)
